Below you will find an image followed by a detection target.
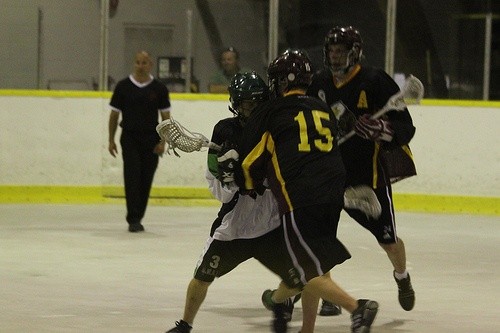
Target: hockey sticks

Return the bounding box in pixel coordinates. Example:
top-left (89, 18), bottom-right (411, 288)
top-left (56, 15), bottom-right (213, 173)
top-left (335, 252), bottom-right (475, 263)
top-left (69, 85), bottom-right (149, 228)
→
top-left (341, 185), bottom-right (384, 219)
top-left (156, 119), bottom-right (224, 154)
top-left (336, 75), bottom-right (424, 146)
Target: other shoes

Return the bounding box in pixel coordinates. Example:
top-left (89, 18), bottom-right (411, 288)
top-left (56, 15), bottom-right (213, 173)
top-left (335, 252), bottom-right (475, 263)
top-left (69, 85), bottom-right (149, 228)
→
top-left (129, 224), bottom-right (143, 232)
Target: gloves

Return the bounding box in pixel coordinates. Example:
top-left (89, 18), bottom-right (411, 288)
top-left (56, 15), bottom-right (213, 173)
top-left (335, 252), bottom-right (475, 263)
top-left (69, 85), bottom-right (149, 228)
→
top-left (352, 114), bottom-right (385, 141)
top-left (239, 185), bottom-right (266, 200)
top-left (215, 140), bottom-right (239, 174)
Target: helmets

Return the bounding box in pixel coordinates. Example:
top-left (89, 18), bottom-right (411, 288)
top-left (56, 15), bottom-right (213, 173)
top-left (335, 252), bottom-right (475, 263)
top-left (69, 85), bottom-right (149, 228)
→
top-left (268, 48), bottom-right (314, 93)
top-left (227, 71), bottom-right (267, 121)
top-left (321, 25), bottom-right (364, 79)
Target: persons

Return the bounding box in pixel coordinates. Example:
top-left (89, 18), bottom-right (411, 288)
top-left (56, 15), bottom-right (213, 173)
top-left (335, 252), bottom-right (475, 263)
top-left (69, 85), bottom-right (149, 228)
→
top-left (166, 69), bottom-right (304, 333)
top-left (108, 50), bottom-right (171, 232)
top-left (207, 46), bottom-right (252, 93)
top-left (241, 49), bottom-right (378, 333)
top-left (304, 26), bottom-right (418, 316)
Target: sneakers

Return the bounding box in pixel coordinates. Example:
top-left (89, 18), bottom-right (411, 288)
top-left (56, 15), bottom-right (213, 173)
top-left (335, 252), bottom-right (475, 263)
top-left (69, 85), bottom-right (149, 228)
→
top-left (349, 298), bottom-right (378, 333)
top-left (166, 319), bottom-right (191, 333)
top-left (261, 288), bottom-right (292, 321)
top-left (319, 299), bottom-right (341, 315)
top-left (393, 269), bottom-right (415, 311)
top-left (272, 299), bottom-right (293, 333)
